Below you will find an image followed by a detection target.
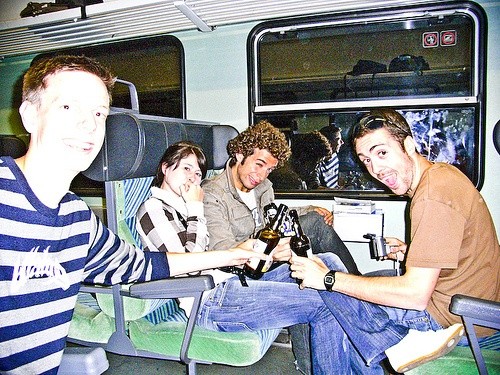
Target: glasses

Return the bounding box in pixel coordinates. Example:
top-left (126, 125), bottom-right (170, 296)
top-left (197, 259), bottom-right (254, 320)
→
top-left (353, 115), bottom-right (410, 142)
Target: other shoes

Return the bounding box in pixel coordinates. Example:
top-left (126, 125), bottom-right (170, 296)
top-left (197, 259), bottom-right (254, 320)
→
top-left (385, 323), bottom-right (465, 373)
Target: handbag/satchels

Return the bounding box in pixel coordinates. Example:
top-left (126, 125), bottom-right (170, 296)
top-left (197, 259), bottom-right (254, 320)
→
top-left (344, 60), bottom-right (387, 97)
top-left (388, 54), bottom-right (431, 76)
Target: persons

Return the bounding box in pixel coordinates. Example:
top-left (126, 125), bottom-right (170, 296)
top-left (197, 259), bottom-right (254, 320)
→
top-left (199, 120), bottom-right (404, 375)
top-left (135, 142), bottom-right (465, 375)
top-left (0, 53), bottom-right (281, 375)
top-left (315, 125), bottom-right (345, 190)
top-left (287, 108), bottom-right (499, 375)
top-left (267, 129), bottom-right (331, 191)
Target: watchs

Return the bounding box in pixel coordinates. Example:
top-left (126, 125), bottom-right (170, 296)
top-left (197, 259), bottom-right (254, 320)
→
top-left (324, 269), bottom-right (336, 292)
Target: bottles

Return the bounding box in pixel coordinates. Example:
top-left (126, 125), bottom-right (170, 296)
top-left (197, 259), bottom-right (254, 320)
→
top-left (262, 202), bottom-right (285, 238)
top-left (289, 209), bottom-right (313, 285)
top-left (241, 205), bottom-right (288, 281)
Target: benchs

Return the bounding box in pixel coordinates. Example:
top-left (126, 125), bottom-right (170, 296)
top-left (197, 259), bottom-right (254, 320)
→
top-left (403, 122), bottom-right (500, 375)
top-left (66, 113), bottom-right (282, 375)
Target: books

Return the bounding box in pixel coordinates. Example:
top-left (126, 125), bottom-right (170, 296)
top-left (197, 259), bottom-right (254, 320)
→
top-left (333, 195), bottom-right (376, 214)
